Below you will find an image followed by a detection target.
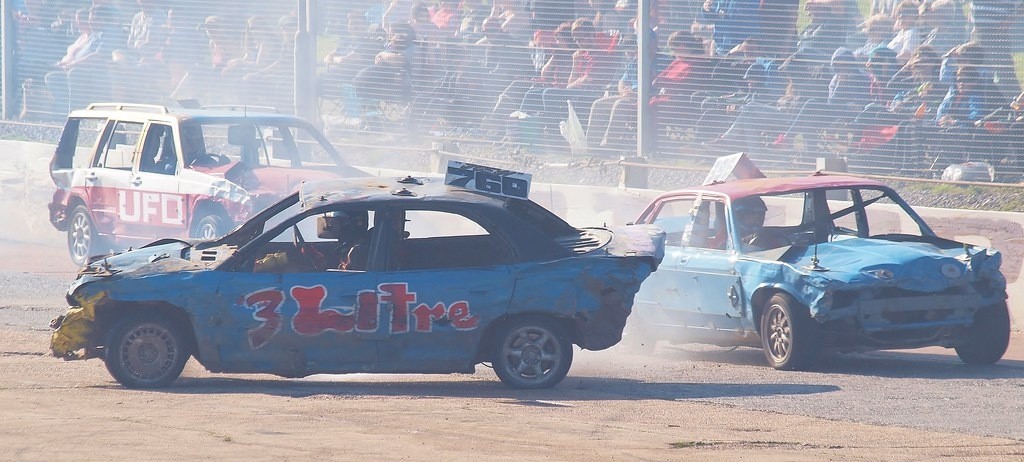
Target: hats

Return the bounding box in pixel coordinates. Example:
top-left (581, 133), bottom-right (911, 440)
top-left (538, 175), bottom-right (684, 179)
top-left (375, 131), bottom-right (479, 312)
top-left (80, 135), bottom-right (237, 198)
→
top-left (831, 46), bottom-right (854, 61)
top-left (743, 63), bottom-right (768, 79)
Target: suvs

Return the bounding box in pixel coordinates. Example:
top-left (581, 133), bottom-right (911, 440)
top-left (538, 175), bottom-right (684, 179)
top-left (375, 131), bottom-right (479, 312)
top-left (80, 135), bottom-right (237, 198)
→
top-left (47, 102), bottom-right (378, 266)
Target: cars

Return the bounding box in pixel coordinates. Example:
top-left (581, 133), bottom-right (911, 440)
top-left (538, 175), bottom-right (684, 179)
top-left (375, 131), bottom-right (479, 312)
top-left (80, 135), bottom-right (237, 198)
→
top-left (620, 171), bottom-right (1010, 370)
top-left (50, 175), bottom-right (666, 390)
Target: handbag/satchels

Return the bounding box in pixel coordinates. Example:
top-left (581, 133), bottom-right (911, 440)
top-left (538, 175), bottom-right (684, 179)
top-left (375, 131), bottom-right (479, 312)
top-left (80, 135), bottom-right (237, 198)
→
top-left (941, 162), bottom-right (995, 183)
top-left (559, 99), bottom-right (588, 155)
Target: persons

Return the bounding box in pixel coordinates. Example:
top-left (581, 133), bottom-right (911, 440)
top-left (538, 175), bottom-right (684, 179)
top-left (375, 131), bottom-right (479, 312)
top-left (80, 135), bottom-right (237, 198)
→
top-left (0, 0), bottom-right (1024, 168)
top-left (707, 196), bottom-right (786, 254)
top-left (302, 208), bottom-right (367, 271)
top-left (157, 126), bottom-right (231, 172)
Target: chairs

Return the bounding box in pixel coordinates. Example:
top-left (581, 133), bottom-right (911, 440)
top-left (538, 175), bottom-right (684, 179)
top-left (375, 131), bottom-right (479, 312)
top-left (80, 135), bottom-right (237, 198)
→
top-left (362, 210), bottom-right (404, 269)
top-left (705, 216), bottom-right (729, 250)
top-left (0, 0), bottom-right (1024, 176)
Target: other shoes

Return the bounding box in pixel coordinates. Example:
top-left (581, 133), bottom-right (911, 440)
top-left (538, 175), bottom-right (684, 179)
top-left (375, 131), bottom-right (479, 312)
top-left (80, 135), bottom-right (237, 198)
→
top-left (701, 139), bottom-right (730, 150)
top-left (766, 134), bottom-right (794, 149)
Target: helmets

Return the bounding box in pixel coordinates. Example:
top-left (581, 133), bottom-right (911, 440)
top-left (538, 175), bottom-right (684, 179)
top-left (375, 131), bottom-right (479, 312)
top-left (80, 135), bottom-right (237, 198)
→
top-left (332, 207), bottom-right (368, 240)
top-left (736, 196), bottom-right (767, 236)
top-left (170, 127), bottom-right (200, 158)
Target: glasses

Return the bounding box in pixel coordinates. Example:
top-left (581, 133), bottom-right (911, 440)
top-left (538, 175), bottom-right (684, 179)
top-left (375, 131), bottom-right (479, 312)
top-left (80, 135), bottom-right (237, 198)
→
top-left (481, 29), bottom-right (494, 33)
top-left (957, 78), bottom-right (971, 83)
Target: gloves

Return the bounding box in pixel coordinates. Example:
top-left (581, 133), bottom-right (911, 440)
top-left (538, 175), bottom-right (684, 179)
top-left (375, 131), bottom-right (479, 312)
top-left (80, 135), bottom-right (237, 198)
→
top-left (302, 245), bottom-right (327, 268)
